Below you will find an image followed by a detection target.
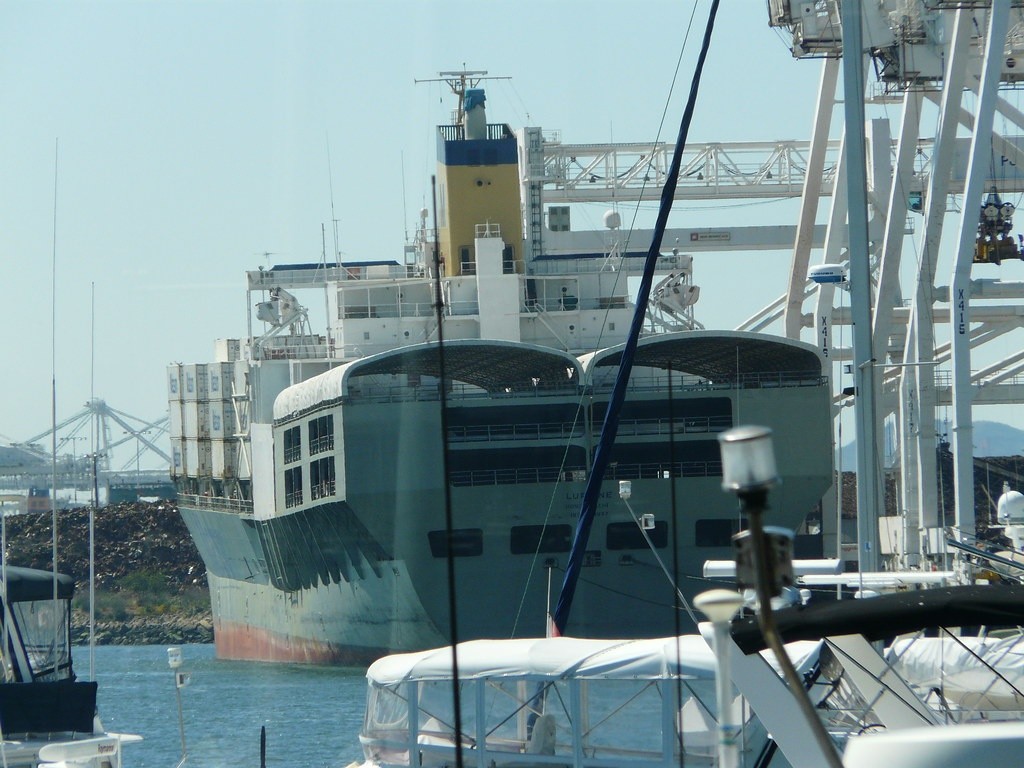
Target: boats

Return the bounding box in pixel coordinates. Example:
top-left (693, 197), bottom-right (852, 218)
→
top-left (354, 1), bottom-right (1024, 768)
top-left (0, 131), bottom-right (146, 768)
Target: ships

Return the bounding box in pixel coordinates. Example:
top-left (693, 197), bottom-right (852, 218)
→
top-left (162, 62), bottom-right (838, 674)
